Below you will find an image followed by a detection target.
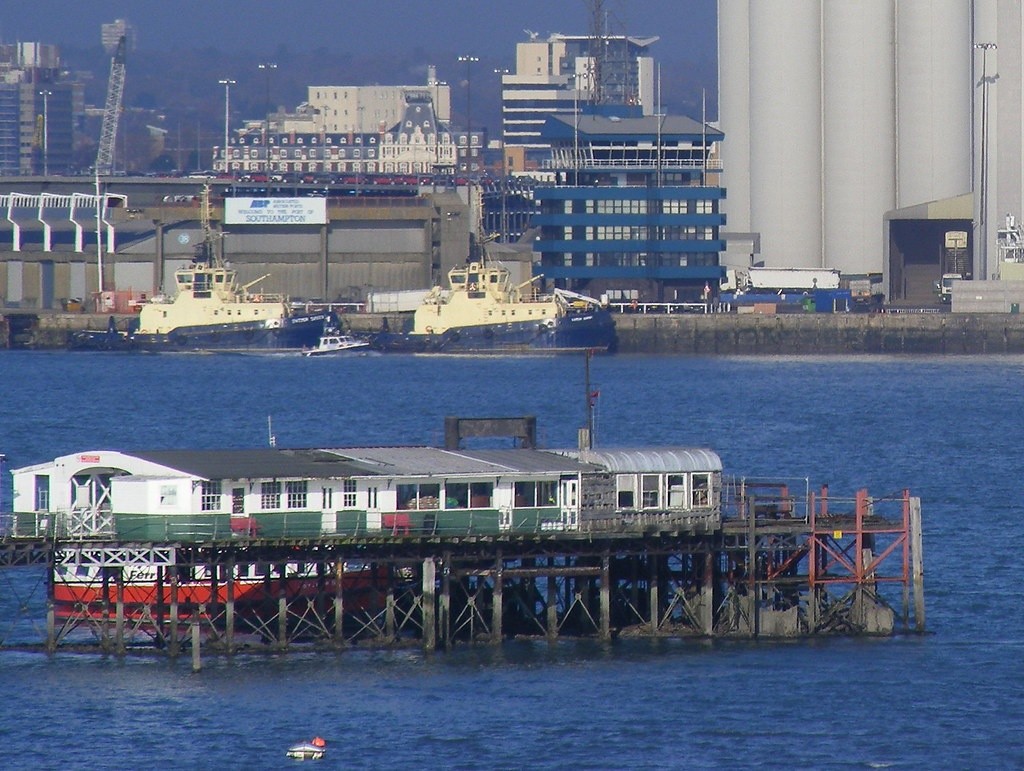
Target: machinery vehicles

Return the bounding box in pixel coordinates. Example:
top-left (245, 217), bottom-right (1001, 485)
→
top-left (719, 267), bottom-right (840, 292)
top-left (940, 248), bottom-right (968, 302)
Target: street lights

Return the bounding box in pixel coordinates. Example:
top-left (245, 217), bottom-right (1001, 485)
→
top-left (219, 78), bottom-right (235, 174)
top-left (458, 55), bottom-right (479, 173)
top-left (39, 90), bottom-right (53, 176)
top-left (258, 62), bottom-right (277, 170)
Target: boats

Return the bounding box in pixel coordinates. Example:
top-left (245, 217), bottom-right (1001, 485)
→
top-left (67, 183), bottom-right (615, 358)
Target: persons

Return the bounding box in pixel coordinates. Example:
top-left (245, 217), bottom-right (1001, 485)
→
top-left (312, 734), bottom-right (326, 746)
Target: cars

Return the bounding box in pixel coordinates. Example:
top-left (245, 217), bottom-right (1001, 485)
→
top-left (147, 171), bottom-right (539, 185)
